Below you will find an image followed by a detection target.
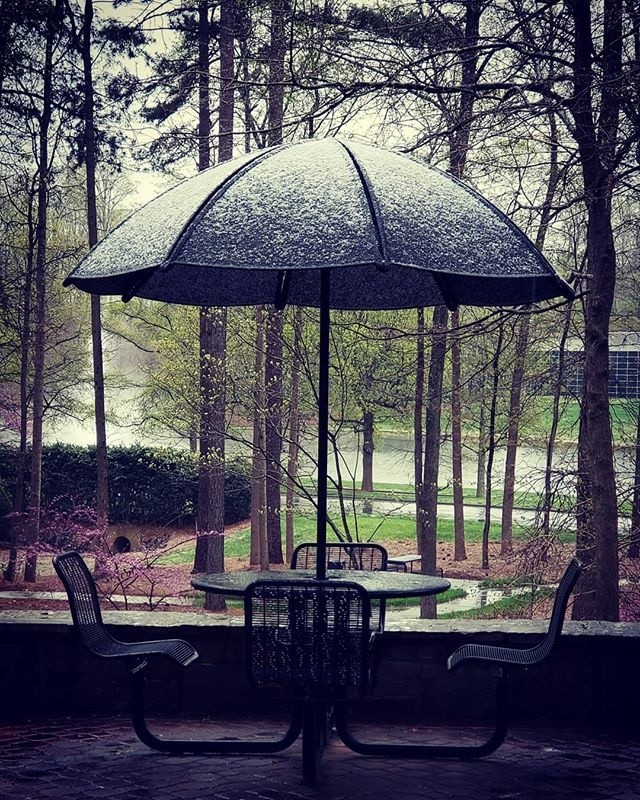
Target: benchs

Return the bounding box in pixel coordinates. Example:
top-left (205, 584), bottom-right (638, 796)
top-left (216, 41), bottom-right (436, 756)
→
top-left (386, 554), bottom-right (422, 572)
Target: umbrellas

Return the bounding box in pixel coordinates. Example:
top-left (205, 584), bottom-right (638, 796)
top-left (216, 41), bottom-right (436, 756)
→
top-left (63, 136), bottom-right (577, 579)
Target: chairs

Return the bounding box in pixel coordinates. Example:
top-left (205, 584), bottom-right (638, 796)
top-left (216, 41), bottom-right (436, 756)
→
top-left (446, 557), bottom-right (586, 761)
top-left (242, 577), bottom-right (370, 768)
top-left (50, 551), bottom-right (200, 756)
top-left (289, 541), bottom-right (390, 732)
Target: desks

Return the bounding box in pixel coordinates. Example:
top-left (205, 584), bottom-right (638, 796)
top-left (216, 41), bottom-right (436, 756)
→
top-left (191, 569), bottom-right (450, 769)
